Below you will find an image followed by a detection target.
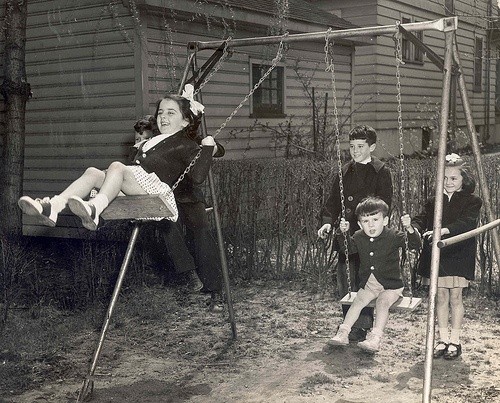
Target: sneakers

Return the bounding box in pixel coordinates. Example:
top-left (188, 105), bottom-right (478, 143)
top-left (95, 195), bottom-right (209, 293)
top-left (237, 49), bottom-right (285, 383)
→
top-left (18, 194), bottom-right (58, 226)
top-left (66, 195), bottom-right (101, 231)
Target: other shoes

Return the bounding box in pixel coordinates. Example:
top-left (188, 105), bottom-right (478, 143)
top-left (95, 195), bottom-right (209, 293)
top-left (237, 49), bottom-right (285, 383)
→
top-left (348, 328), bottom-right (367, 342)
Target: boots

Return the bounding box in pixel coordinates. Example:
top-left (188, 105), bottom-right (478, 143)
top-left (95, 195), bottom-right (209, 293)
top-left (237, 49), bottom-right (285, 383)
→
top-left (328, 324), bottom-right (350, 346)
top-left (356, 329), bottom-right (384, 352)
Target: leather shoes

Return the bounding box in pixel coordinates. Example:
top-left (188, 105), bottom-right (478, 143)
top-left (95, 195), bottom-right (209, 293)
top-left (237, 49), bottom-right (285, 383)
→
top-left (184, 269), bottom-right (204, 292)
top-left (209, 292), bottom-right (225, 314)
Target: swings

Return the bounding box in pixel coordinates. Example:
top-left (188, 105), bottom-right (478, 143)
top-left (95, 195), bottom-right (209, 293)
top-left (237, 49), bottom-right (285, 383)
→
top-left (99, 31), bottom-right (289, 220)
top-left (324, 20), bottom-right (423, 312)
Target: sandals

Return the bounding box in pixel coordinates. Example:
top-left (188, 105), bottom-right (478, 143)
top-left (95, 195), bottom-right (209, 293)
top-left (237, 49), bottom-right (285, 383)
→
top-left (433, 342), bottom-right (463, 359)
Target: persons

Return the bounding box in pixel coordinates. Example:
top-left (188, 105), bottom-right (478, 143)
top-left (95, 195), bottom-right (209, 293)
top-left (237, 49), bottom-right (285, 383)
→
top-left (411, 153), bottom-right (483, 359)
top-left (317, 125), bottom-right (393, 342)
top-left (133, 115), bottom-right (224, 314)
top-left (327, 196), bottom-right (421, 352)
top-left (18, 84), bottom-right (216, 231)
